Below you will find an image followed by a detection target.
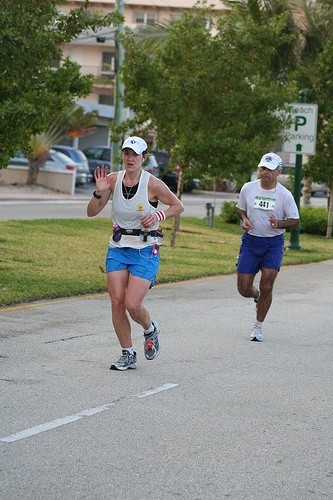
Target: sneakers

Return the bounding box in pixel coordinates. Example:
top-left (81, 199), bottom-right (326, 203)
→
top-left (110, 350), bottom-right (137, 371)
top-left (143, 322), bottom-right (160, 360)
top-left (250, 320), bottom-right (264, 341)
top-left (254, 289), bottom-right (260, 311)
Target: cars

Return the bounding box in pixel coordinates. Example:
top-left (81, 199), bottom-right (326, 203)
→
top-left (9, 145), bottom-right (193, 194)
top-left (276, 163), bottom-right (330, 195)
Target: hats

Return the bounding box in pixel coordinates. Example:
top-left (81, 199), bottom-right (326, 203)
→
top-left (258, 153), bottom-right (282, 171)
top-left (121, 136), bottom-right (147, 155)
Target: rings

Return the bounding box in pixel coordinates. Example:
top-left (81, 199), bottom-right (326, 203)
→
top-left (272, 223), bottom-right (274, 225)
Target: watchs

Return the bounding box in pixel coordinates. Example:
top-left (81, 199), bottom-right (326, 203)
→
top-left (93, 190), bottom-right (101, 199)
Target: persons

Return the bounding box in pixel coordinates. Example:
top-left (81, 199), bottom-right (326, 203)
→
top-left (236, 151), bottom-right (301, 342)
top-left (86, 136), bottom-right (185, 371)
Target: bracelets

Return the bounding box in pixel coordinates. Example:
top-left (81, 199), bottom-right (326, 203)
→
top-left (242, 218), bottom-right (247, 222)
top-left (154, 210), bottom-right (165, 222)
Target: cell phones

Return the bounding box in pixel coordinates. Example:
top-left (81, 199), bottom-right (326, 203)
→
top-left (152, 243), bottom-right (160, 259)
